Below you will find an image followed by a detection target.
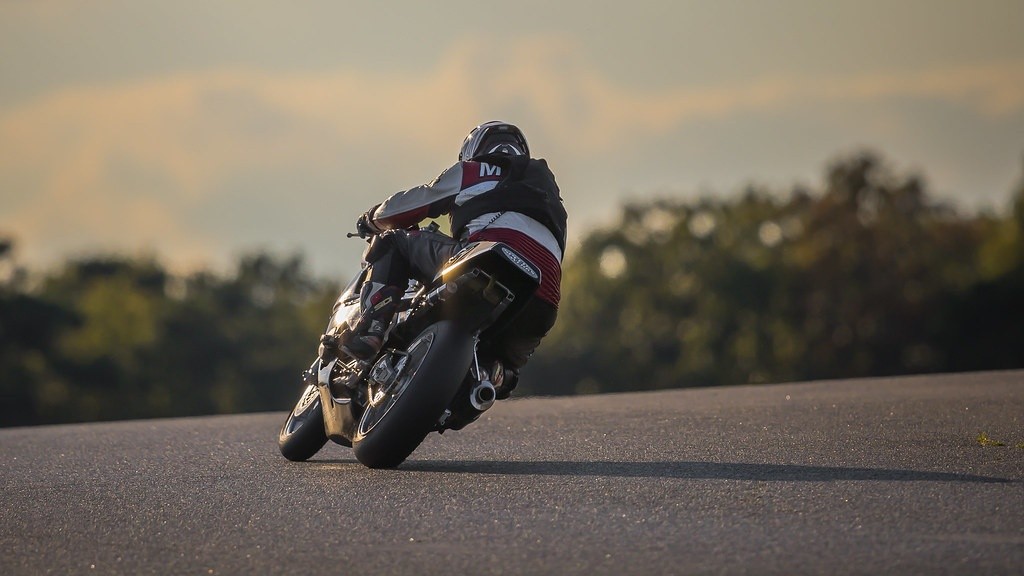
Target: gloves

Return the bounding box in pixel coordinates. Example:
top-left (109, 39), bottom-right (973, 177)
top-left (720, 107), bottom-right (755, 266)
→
top-left (355, 201), bottom-right (383, 240)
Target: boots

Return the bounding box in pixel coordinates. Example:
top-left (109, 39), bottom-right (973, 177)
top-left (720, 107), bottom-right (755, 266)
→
top-left (319, 280), bottom-right (407, 362)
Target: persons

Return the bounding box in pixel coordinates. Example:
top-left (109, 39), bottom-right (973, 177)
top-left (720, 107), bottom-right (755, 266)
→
top-left (338, 120), bottom-right (568, 373)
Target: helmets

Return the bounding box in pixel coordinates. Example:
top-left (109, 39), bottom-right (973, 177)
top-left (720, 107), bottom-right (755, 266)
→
top-left (459, 119), bottom-right (530, 163)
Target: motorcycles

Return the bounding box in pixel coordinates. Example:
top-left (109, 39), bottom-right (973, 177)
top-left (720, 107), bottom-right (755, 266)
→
top-left (277, 221), bottom-right (543, 470)
